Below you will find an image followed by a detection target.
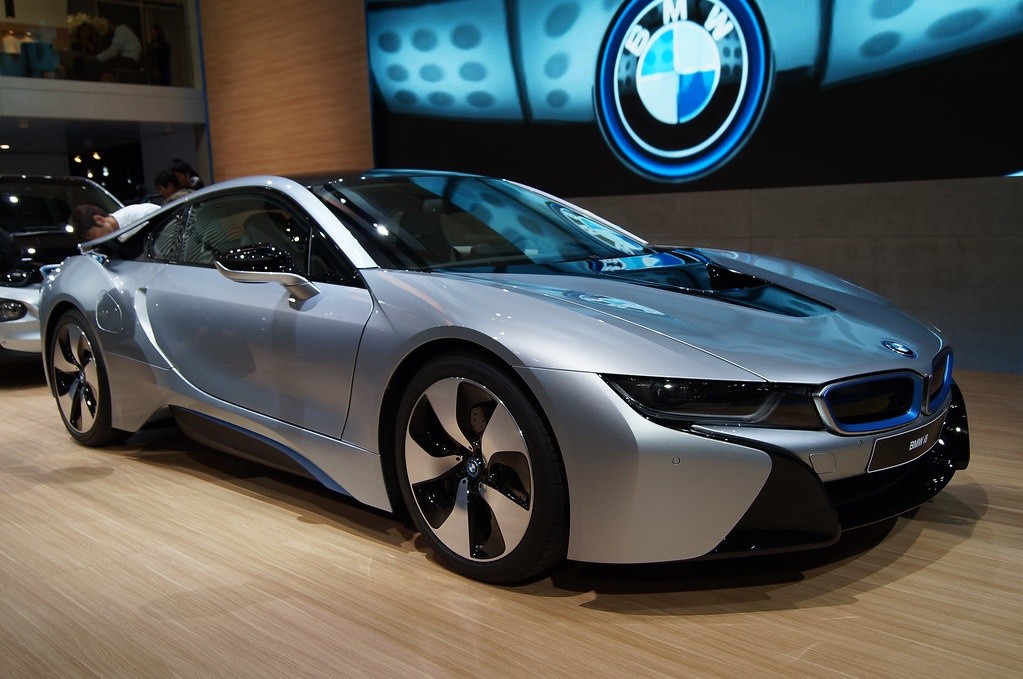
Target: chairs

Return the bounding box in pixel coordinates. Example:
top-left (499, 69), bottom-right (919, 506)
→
top-left (394, 209), bottom-right (462, 265)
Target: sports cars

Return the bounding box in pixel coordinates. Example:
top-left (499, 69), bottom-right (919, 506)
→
top-left (35, 162), bottom-right (975, 592)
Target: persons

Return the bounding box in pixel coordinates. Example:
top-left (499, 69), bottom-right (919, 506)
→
top-left (68, 203), bottom-right (162, 242)
top-left (155, 158), bottom-right (204, 208)
top-left (70, 12), bottom-right (171, 86)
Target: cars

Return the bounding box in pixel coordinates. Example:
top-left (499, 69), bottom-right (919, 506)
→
top-left (0, 174), bottom-right (125, 374)
